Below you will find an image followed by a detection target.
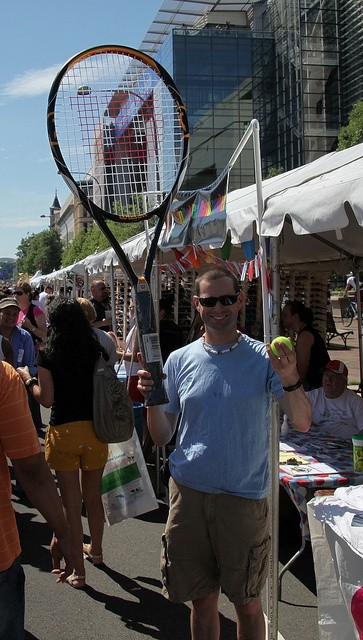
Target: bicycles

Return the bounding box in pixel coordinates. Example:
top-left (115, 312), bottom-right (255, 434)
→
top-left (340, 295), bottom-right (362, 328)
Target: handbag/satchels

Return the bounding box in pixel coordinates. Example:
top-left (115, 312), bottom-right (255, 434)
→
top-left (99, 429), bottom-right (158, 527)
top-left (92, 354), bottom-right (134, 444)
top-left (20, 315), bottom-right (42, 345)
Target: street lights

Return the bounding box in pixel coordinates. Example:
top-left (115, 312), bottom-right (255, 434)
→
top-left (77, 85), bottom-right (161, 223)
top-left (57, 170), bottom-right (103, 210)
top-left (39, 214), bottom-right (69, 248)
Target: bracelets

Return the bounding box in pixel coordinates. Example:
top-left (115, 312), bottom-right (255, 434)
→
top-left (25, 377), bottom-right (37, 391)
top-left (281, 378), bottom-right (303, 393)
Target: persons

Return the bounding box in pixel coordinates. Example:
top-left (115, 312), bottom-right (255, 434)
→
top-left (115, 297), bottom-right (149, 446)
top-left (74, 297), bottom-right (117, 373)
top-left (12, 282), bottom-right (47, 439)
top-left (87, 279), bottom-right (112, 333)
top-left (0, 297), bottom-right (38, 378)
top-left (327, 291), bottom-right (338, 334)
top-left (344, 271), bottom-right (363, 320)
top-left (282, 299), bottom-right (332, 393)
top-left (0, 280), bottom-right (71, 314)
top-left (172, 284), bottom-right (192, 326)
top-left (136, 264), bottom-right (313, 640)
top-left (303, 360), bottom-right (363, 440)
top-left (16, 295), bottom-right (111, 588)
top-left (0, 360), bottom-right (76, 640)
top-left (157, 298), bottom-right (184, 369)
top-left (237, 285), bottom-right (257, 329)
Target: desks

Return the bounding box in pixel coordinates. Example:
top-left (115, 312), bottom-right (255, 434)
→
top-left (280, 423), bottom-right (362, 600)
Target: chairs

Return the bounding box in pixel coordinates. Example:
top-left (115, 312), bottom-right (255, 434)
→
top-left (327, 299), bottom-right (354, 349)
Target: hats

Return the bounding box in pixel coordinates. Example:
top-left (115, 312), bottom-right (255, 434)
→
top-left (0, 297), bottom-right (20, 311)
top-left (322, 359), bottom-right (348, 378)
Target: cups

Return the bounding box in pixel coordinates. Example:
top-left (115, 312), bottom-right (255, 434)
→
top-left (352, 434), bottom-right (363, 475)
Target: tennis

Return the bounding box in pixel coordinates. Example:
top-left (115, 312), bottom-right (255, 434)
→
top-left (270, 337), bottom-right (292, 358)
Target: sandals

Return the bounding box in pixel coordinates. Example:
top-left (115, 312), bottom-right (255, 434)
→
top-left (82, 543), bottom-right (103, 565)
top-left (66, 573), bottom-right (86, 588)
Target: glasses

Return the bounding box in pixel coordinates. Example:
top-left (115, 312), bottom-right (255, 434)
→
top-left (12, 291), bottom-right (23, 296)
top-left (197, 292), bottom-right (239, 306)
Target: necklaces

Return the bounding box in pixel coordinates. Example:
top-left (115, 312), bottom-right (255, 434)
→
top-left (201, 330), bottom-right (242, 355)
